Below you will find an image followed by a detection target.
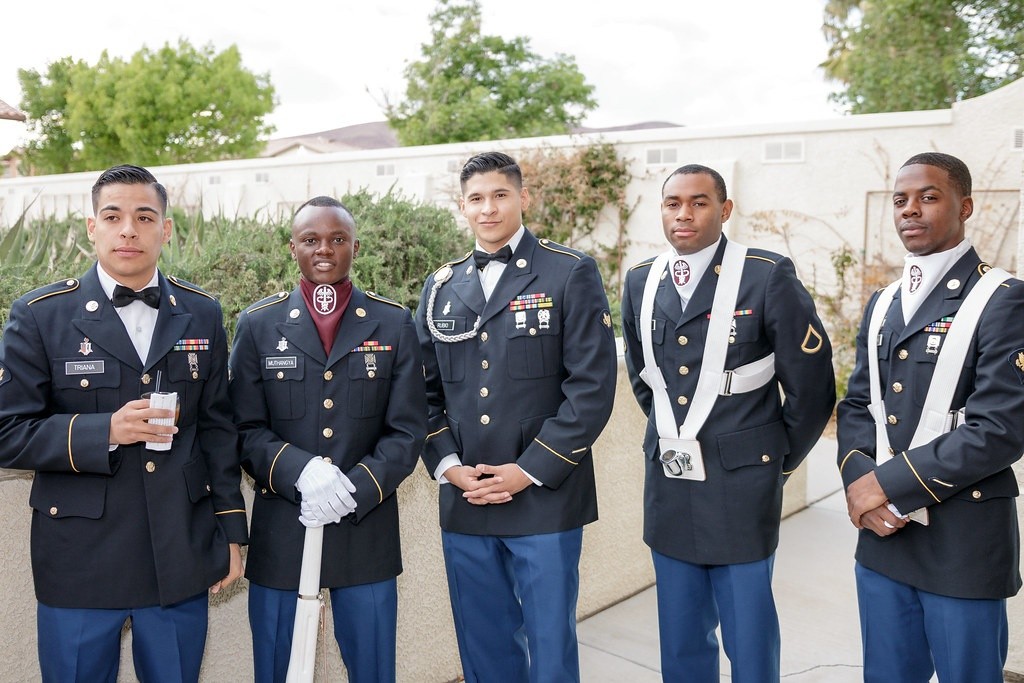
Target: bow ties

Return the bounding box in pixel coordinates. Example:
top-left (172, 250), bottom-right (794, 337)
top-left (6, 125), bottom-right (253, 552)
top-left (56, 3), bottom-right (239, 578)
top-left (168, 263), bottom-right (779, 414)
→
top-left (473, 244), bottom-right (512, 272)
top-left (113, 284), bottom-right (160, 310)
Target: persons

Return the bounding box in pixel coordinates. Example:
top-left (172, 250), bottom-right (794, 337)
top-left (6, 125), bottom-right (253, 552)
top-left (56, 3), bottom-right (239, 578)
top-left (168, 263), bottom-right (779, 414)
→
top-left (1, 163), bottom-right (248, 683)
top-left (836, 152), bottom-right (1023, 683)
top-left (228, 195), bottom-right (430, 683)
top-left (620, 162), bottom-right (837, 683)
top-left (414, 152), bottom-right (619, 683)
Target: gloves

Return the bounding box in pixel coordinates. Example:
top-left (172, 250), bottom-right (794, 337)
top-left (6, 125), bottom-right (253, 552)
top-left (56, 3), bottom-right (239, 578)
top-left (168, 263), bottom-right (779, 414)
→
top-left (295, 456), bottom-right (358, 528)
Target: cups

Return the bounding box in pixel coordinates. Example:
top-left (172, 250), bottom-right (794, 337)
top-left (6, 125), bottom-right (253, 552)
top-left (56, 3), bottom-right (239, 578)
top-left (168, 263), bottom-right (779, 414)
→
top-left (141, 391), bottom-right (180, 426)
